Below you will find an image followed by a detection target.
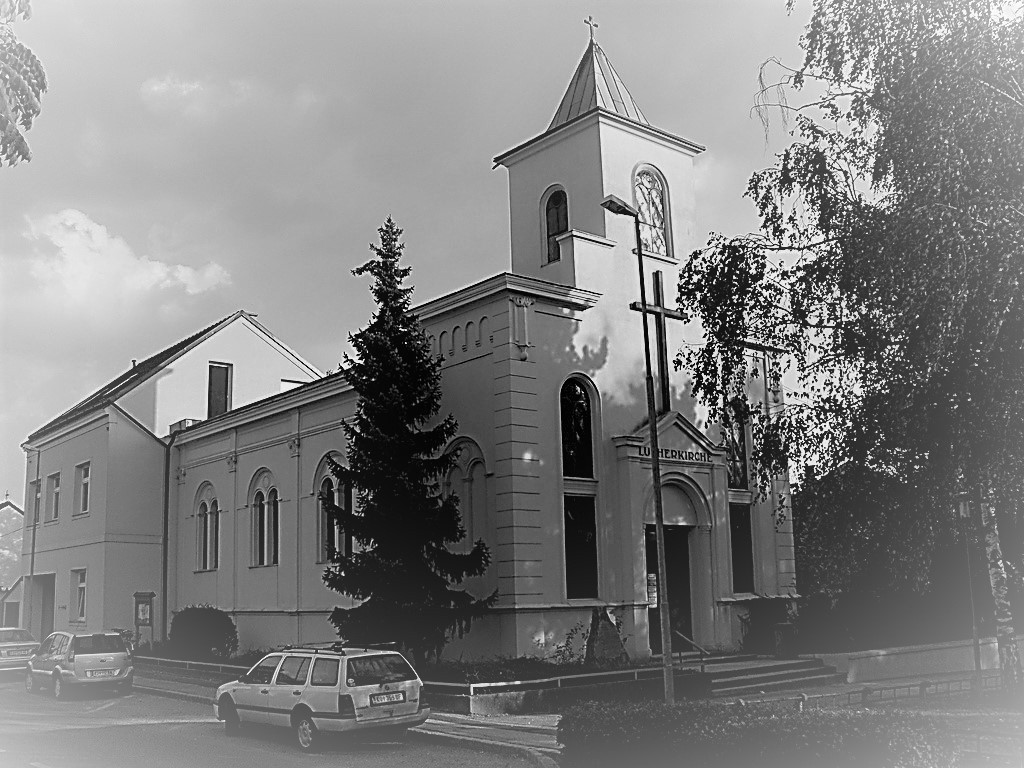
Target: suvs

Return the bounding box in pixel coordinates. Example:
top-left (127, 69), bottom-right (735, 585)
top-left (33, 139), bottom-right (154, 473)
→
top-left (213, 640), bottom-right (431, 752)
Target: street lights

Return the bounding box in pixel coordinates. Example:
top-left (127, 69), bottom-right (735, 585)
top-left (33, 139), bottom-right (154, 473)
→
top-left (22, 443), bottom-right (41, 635)
top-left (599, 194), bottom-right (676, 704)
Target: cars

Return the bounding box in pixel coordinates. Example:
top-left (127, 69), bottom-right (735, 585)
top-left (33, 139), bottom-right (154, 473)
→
top-left (22, 629), bottom-right (133, 699)
top-left (0, 627), bottom-right (41, 672)
